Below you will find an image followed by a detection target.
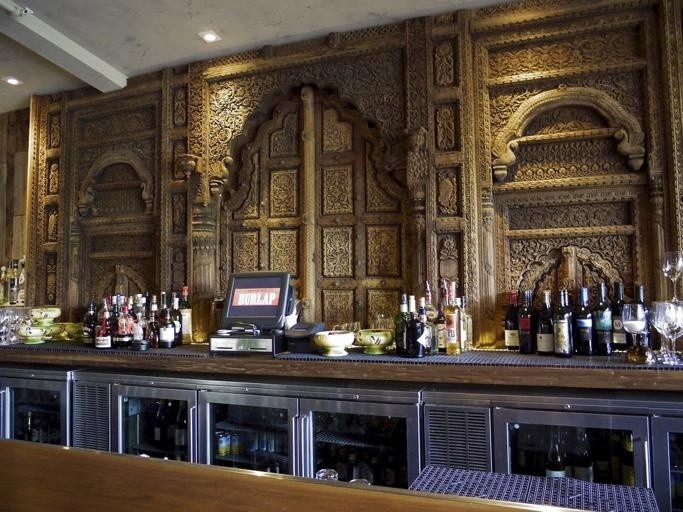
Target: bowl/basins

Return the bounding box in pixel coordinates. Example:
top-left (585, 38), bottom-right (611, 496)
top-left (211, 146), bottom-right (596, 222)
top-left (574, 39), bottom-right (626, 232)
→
top-left (18, 301), bottom-right (83, 345)
top-left (314, 328), bottom-right (394, 357)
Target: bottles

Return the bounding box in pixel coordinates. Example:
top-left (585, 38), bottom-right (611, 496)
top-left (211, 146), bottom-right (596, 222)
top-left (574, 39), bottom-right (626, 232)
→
top-left (24, 409), bottom-right (47, 442)
top-left (326, 445), bottom-right (397, 486)
top-left (518, 428), bottom-right (634, 485)
top-left (505, 281), bottom-right (651, 357)
top-left (81, 287), bottom-right (194, 351)
top-left (152, 399), bottom-right (189, 449)
top-left (0, 254), bottom-right (26, 304)
top-left (393, 278), bottom-right (472, 358)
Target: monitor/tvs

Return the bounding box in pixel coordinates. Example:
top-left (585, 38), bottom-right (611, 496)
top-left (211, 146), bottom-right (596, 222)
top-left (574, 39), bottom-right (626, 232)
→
top-left (222, 272), bottom-right (295, 329)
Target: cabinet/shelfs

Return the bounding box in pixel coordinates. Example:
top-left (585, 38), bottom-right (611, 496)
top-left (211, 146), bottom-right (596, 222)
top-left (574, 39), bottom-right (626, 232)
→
top-left (198, 390), bottom-right (422, 489)
top-left (0, 376), bottom-right (72, 447)
top-left (493, 406), bottom-right (683, 512)
top-left (111, 384), bottom-right (199, 465)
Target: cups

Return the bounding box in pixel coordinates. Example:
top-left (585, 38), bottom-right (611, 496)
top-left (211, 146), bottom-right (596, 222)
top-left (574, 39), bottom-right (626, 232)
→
top-left (315, 468), bottom-right (370, 487)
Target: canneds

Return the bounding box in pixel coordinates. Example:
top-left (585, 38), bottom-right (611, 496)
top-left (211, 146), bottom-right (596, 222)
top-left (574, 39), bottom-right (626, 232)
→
top-left (215, 431), bottom-right (242, 457)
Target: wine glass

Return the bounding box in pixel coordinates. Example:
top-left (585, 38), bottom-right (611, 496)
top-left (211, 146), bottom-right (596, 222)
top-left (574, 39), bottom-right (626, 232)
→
top-left (0, 308), bottom-right (23, 348)
top-left (621, 252), bottom-right (683, 368)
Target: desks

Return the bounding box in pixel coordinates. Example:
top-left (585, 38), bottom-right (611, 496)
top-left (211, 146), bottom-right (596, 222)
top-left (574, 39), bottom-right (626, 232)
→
top-left (0, 437), bottom-right (585, 512)
